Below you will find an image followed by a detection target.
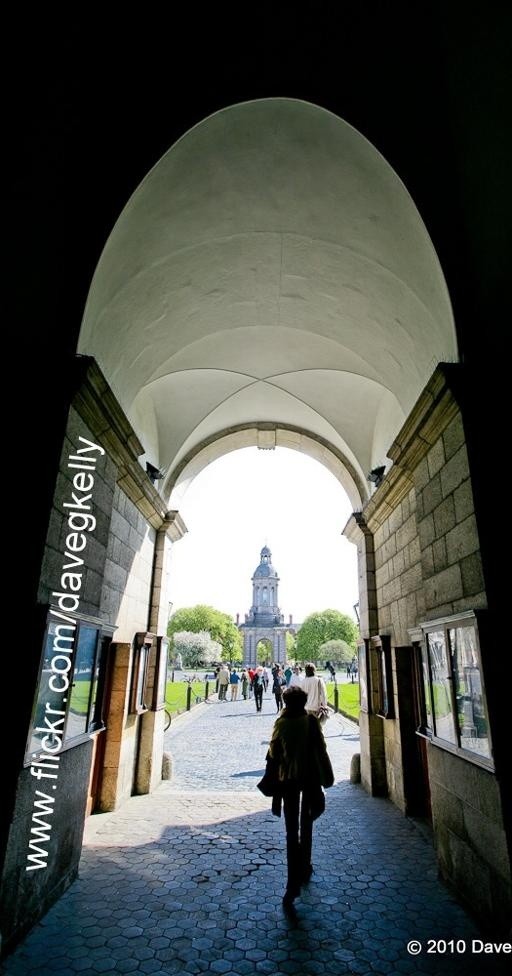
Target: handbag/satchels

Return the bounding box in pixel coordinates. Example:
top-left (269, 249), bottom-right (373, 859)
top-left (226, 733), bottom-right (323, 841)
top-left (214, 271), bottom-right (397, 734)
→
top-left (317, 705), bottom-right (329, 723)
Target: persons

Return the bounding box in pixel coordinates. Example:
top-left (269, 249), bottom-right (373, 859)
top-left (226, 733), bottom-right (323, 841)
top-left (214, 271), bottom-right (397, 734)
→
top-left (213, 662), bottom-right (302, 713)
top-left (257, 686), bottom-right (334, 904)
top-left (300, 663), bottom-right (327, 723)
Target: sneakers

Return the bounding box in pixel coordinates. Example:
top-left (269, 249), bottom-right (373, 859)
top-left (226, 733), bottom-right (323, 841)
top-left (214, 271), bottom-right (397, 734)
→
top-left (281, 886), bottom-right (303, 907)
